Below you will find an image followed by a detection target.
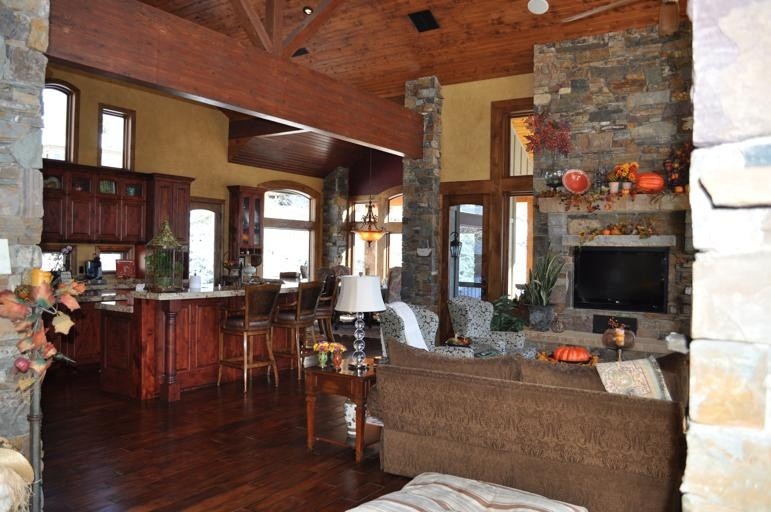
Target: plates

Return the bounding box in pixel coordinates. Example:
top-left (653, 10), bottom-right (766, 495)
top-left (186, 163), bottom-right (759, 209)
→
top-left (444, 337), bottom-right (472, 347)
top-left (563, 169), bottom-right (591, 194)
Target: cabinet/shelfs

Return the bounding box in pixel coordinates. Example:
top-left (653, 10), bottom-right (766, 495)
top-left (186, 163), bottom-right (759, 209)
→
top-left (42, 157), bottom-right (95, 244)
top-left (226, 184), bottom-right (268, 268)
top-left (148, 172), bottom-right (196, 245)
top-left (95, 165), bottom-right (147, 245)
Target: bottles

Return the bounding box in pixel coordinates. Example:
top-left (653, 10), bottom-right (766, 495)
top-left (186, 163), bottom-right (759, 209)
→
top-left (245, 249), bottom-right (252, 267)
top-left (239, 250), bottom-right (245, 268)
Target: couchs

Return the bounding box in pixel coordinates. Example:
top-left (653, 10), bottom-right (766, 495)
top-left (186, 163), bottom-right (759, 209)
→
top-left (375, 336), bottom-right (689, 512)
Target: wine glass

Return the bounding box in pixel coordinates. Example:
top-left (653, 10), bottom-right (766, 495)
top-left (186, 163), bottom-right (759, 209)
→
top-left (545, 170), bottom-right (562, 196)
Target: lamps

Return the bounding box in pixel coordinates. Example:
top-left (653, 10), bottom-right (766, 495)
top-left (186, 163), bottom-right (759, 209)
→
top-left (449, 231), bottom-right (463, 257)
top-left (349, 148), bottom-right (390, 249)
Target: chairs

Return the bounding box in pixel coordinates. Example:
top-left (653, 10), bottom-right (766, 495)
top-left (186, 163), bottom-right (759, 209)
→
top-left (381, 302), bottom-right (476, 360)
top-left (449, 295), bottom-right (537, 361)
top-left (216, 265), bottom-right (403, 392)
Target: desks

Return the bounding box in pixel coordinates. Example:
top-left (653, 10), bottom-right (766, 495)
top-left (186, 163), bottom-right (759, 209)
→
top-left (304, 357), bottom-right (380, 462)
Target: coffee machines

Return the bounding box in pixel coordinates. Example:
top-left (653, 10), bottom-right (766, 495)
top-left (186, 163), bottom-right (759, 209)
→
top-left (85, 256), bottom-right (107, 285)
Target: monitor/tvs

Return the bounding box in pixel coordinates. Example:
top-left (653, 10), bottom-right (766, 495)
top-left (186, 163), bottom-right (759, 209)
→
top-left (573, 247), bottom-right (668, 313)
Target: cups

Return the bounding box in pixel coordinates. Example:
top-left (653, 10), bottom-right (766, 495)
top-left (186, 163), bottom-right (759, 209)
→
top-left (622, 181), bottom-right (633, 193)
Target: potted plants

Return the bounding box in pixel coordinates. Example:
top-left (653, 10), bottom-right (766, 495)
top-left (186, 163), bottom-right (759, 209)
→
top-left (515, 240), bottom-right (568, 331)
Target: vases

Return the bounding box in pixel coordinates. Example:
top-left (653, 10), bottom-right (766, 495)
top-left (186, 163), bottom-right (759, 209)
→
top-left (542, 161), bottom-right (568, 191)
top-left (622, 182), bottom-right (633, 189)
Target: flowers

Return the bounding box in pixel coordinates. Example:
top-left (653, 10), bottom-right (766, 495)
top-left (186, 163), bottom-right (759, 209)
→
top-left (614, 161), bottom-right (639, 182)
top-left (607, 171), bottom-right (618, 182)
top-left (522, 106), bottom-right (577, 161)
